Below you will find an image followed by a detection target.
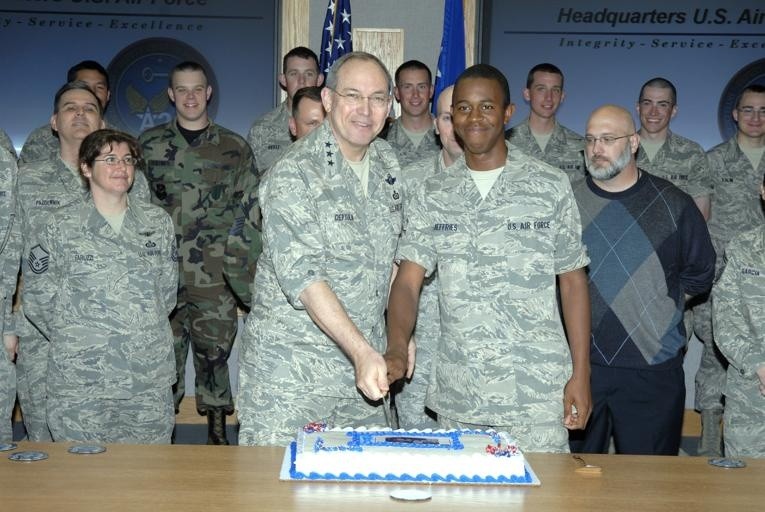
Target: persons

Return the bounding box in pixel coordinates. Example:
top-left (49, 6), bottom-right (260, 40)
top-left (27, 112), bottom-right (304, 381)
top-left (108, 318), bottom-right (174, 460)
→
top-left (628, 77), bottom-right (714, 351)
top-left (235, 46), bottom-right (401, 448)
top-left (1, 60), bottom-right (180, 448)
top-left (136, 61), bottom-right (264, 445)
top-left (382, 59), bottom-right (592, 453)
top-left (695, 84), bottom-right (765, 456)
top-left (551, 105), bottom-right (716, 456)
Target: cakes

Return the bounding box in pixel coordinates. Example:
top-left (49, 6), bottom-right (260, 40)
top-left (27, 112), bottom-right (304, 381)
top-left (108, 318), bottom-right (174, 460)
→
top-left (290, 422), bottom-right (533, 484)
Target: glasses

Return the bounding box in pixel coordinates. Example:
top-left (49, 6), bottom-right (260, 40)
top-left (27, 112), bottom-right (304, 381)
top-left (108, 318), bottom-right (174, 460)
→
top-left (95, 156), bottom-right (138, 165)
top-left (328, 83), bottom-right (388, 106)
top-left (584, 133), bottom-right (634, 145)
top-left (736, 107), bottom-right (764, 115)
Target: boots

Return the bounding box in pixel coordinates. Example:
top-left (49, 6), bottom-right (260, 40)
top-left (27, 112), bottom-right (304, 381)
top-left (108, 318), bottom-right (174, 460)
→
top-left (695, 408), bottom-right (722, 457)
top-left (207, 407), bottom-right (230, 445)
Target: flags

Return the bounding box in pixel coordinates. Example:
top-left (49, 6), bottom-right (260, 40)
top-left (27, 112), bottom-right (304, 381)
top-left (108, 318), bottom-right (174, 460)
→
top-left (431, 0), bottom-right (466, 115)
top-left (320, 0), bottom-right (354, 74)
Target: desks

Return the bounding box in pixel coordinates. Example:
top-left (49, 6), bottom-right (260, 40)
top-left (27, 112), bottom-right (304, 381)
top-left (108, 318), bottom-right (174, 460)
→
top-left (0, 442), bottom-right (764, 511)
